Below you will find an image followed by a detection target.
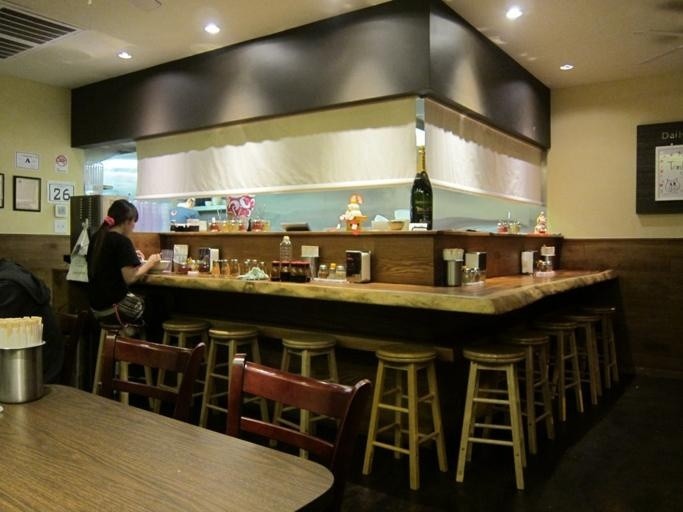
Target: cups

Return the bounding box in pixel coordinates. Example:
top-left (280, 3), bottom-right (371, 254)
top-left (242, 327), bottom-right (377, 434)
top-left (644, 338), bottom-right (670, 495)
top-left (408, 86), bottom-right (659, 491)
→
top-left (161, 248), bottom-right (172, 273)
top-left (303, 256), bottom-right (319, 277)
top-left (440, 260), bottom-right (463, 286)
top-left (0, 342), bottom-right (50, 405)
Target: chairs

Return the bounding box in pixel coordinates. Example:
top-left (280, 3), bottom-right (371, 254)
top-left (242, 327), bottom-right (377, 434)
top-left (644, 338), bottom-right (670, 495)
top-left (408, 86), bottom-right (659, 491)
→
top-left (223, 350), bottom-right (374, 512)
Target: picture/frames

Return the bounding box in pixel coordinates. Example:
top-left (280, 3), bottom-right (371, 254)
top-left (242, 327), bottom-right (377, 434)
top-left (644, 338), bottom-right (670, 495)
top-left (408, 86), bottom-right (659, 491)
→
top-left (13, 174), bottom-right (42, 214)
top-left (1, 173), bottom-right (6, 208)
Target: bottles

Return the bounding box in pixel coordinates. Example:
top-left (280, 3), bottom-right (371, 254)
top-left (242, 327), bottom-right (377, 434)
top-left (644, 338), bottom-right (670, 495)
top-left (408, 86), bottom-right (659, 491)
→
top-left (317, 263), bottom-right (345, 279)
top-left (496, 221), bottom-right (520, 233)
top-left (278, 236), bottom-right (292, 263)
top-left (208, 216), bottom-right (269, 231)
top-left (211, 259), bottom-right (266, 278)
top-left (410, 146), bottom-right (432, 229)
top-left (270, 262), bottom-right (310, 281)
top-left (461, 266), bottom-right (485, 283)
top-left (535, 260), bottom-right (553, 272)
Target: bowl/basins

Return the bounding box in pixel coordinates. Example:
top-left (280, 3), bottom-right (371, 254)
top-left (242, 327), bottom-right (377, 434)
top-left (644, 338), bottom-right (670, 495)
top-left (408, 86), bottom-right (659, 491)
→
top-left (139, 261), bottom-right (170, 273)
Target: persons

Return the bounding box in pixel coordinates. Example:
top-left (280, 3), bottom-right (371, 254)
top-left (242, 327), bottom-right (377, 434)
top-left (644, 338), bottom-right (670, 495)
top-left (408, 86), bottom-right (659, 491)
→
top-left (1, 253), bottom-right (65, 385)
top-left (81, 198), bottom-right (164, 337)
top-left (168, 195), bottom-right (200, 225)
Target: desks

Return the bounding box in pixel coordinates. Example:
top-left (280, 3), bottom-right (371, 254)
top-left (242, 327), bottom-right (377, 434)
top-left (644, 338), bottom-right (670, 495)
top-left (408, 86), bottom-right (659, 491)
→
top-left (0, 382), bottom-right (338, 512)
